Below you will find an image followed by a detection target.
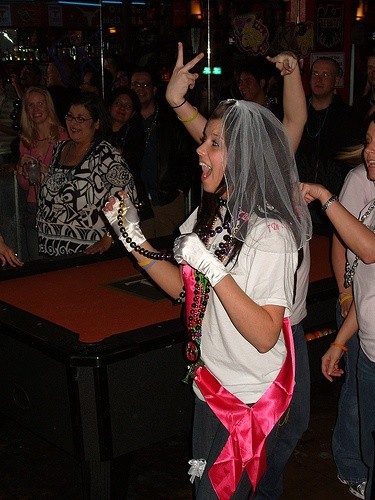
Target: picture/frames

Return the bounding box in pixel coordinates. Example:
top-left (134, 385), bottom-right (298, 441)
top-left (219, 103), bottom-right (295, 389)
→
top-left (0, 4), bottom-right (12, 27)
top-left (47, 4), bottom-right (64, 27)
top-left (309, 52), bottom-right (345, 89)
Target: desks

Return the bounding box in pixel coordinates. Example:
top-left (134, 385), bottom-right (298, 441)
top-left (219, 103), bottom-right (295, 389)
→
top-left (0, 232), bottom-right (335, 500)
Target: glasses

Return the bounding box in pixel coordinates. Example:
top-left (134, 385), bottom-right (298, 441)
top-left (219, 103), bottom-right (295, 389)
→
top-left (65, 113), bottom-right (94, 123)
top-left (131, 81), bottom-right (152, 87)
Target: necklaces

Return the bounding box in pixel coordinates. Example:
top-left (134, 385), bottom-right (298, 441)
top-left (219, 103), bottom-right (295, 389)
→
top-left (344, 199), bottom-right (375, 288)
top-left (114, 193), bottom-right (269, 383)
top-left (38, 138), bottom-right (46, 141)
top-left (305, 100), bottom-right (329, 137)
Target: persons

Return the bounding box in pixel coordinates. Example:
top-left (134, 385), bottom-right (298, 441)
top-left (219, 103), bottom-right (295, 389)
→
top-left (355, 51), bottom-right (375, 121)
top-left (0, 62), bottom-right (137, 266)
top-left (214, 51), bottom-right (249, 106)
top-left (294, 58), bottom-right (362, 237)
top-left (167, 42), bottom-right (306, 153)
top-left (102, 52), bottom-right (216, 239)
top-left (239, 58), bottom-right (284, 122)
top-left (103, 99), bottom-right (312, 500)
top-left (293, 122), bottom-right (375, 500)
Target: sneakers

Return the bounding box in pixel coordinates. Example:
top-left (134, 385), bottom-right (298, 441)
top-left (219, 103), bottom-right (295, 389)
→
top-left (338, 471), bottom-right (367, 499)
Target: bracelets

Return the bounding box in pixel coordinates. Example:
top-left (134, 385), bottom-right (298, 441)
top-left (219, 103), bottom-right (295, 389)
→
top-left (330, 343), bottom-right (348, 351)
top-left (177, 106), bottom-right (198, 122)
top-left (339, 295), bottom-right (353, 304)
top-left (322, 194), bottom-right (337, 213)
top-left (172, 99), bottom-right (187, 108)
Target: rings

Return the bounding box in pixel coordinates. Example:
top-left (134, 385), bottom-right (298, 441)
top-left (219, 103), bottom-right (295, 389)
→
top-left (14, 254), bottom-right (18, 257)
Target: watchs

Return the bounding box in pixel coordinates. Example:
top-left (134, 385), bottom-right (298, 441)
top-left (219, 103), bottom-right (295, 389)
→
top-left (107, 232), bottom-right (114, 238)
